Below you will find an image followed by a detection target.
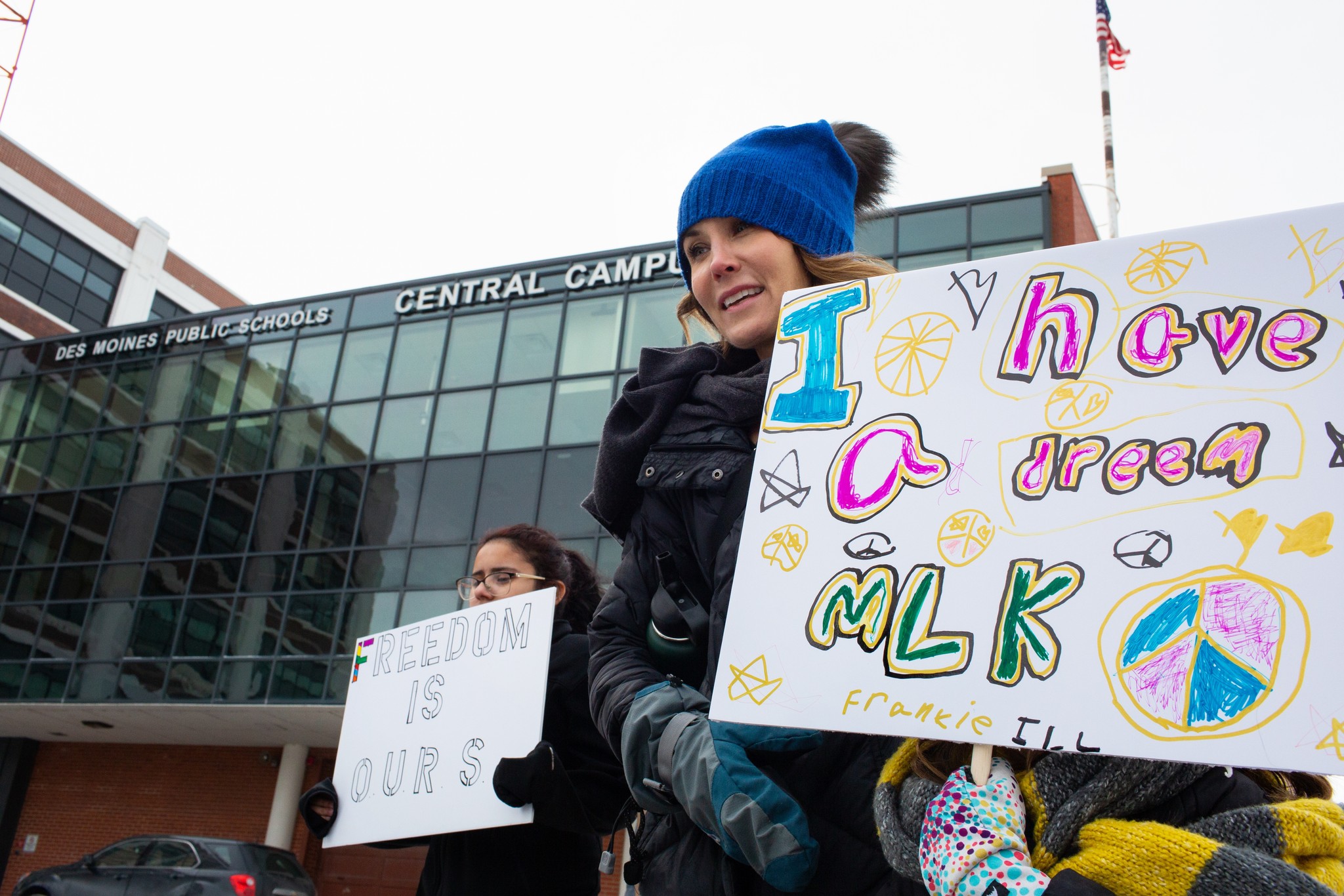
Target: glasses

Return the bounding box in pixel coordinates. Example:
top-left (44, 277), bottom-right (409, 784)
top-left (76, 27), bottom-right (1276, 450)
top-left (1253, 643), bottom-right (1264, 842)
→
top-left (455, 570), bottom-right (547, 601)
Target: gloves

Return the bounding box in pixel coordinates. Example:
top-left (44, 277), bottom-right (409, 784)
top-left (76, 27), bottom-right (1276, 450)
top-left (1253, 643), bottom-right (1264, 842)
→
top-left (624, 675), bottom-right (825, 888)
top-left (919, 758), bottom-right (1051, 896)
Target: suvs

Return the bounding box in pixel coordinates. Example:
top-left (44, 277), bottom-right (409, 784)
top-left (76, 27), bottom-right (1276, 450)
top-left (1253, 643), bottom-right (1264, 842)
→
top-left (12, 834), bottom-right (318, 896)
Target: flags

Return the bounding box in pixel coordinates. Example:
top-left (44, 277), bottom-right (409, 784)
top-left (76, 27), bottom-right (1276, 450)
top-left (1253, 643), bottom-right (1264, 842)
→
top-left (1095, 0), bottom-right (1130, 70)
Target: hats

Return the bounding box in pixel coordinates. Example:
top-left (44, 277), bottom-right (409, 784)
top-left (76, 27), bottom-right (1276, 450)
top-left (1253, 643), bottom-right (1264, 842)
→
top-left (676, 121), bottom-right (859, 290)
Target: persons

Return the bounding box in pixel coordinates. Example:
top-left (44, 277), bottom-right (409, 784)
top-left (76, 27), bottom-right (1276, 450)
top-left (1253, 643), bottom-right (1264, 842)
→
top-left (574, 117), bottom-right (931, 896)
top-left (295, 523), bottom-right (639, 896)
top-left (875, 736), bottom-right (1344, 896)
